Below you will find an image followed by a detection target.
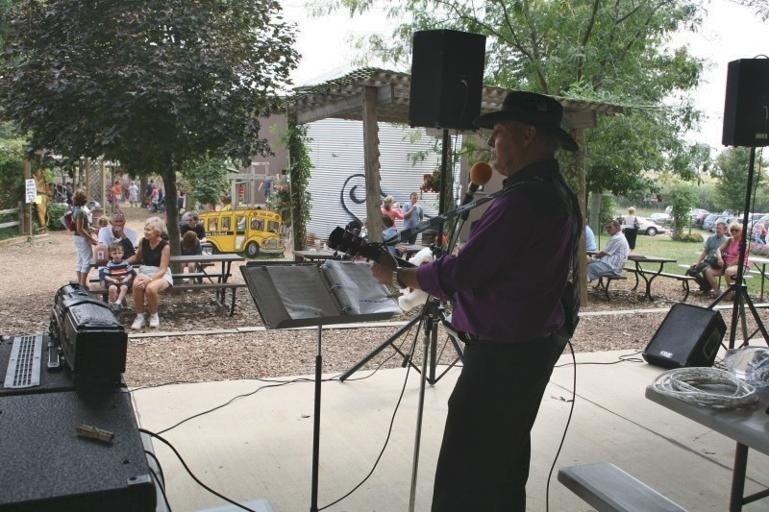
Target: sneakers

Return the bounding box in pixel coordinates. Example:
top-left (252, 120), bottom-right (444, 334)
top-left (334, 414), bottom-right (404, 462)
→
top-left (148, 313), bottom-right (161, 329)
top-left (129, 315), bottom-right (146, 330)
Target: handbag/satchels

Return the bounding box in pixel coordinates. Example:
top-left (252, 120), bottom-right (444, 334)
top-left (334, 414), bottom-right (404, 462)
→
top-left (59, 211), bottom-right (76, 232)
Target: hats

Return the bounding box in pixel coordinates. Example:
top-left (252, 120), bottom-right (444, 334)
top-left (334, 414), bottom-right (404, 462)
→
top-left (469, 89), bottom-right (581, 152)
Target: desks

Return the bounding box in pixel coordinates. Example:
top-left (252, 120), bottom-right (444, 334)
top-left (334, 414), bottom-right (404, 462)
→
top-left (645, 370), bottom-right (769, 511)
top-left (291, 244), bottom-right (428, 262)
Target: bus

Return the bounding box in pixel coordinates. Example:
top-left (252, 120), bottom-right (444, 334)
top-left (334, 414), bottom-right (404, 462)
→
top-left (199, 209), bottom-right (284, 257)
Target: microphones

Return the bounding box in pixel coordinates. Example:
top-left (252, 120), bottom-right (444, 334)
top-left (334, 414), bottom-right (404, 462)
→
top-left (460, 161), bottom-right (492, 219)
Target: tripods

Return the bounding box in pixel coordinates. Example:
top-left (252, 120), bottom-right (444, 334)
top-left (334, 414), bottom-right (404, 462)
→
top-left (706, 147), bottom-right (769, 351)
top-left (341, 131), bottom-right (464, 381)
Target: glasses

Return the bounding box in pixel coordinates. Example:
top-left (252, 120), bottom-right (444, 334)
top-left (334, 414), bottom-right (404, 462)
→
top-left (730, 228), bottom-right (739, 233)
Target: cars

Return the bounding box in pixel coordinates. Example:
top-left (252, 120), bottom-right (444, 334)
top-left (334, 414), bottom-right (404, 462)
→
top-left (645, 205), bottom-right (769, 248)
top-left (613, 215), bottom-right (666, 236)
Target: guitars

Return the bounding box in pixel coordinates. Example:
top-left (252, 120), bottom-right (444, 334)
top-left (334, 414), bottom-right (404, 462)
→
top-left (326, 224), bottom-right (579, 355)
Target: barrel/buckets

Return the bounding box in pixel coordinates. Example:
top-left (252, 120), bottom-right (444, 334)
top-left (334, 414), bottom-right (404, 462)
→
top-left (95, 242), bottom-right (109, 265)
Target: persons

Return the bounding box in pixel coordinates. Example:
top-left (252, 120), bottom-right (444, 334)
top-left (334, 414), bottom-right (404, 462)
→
top-left (705, 222), bottom-right (751, 301)
top-left (71, 180), bottom-right (208, 330)
top-left (624, 207), bottom-right (640, 249)
top-left (586, 218), bottom-right (597, 251)
top-left (586, 220), bottom-right (630, 283)
top-left (687, 222), bottom-right (727, 293)
top-left (380, 196), bottom-right (417, 227)
top-left (382, 214), bottom-right (398, 254)
top-left (400, 192), bottom-right (424, 258)
top-left (370, 91), bottom-right (583, 512)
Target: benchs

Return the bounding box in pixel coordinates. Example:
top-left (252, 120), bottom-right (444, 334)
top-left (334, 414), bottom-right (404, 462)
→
top-left (82, 251), bottom-right (249, 316)
top-left (585, 246), bottom-right (769, 301)
top-left (557, 460), bottom-right (690, 512)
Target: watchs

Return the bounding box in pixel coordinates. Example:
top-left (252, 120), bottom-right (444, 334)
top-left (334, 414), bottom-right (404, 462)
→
top-left (392, 266), bottom-right (406, 289)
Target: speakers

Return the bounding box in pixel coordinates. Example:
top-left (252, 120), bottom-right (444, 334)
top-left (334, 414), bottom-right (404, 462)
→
top-left (721, 59), bottom-right (769, 147)
top-left (0, 335), bottom-right (128, 396)
top-left (0, 386), bottom-right (158, 511)
top-left (410, 29), bottom-right (486, 131)
top-left (641, 303), bottom-right (727, 369)
